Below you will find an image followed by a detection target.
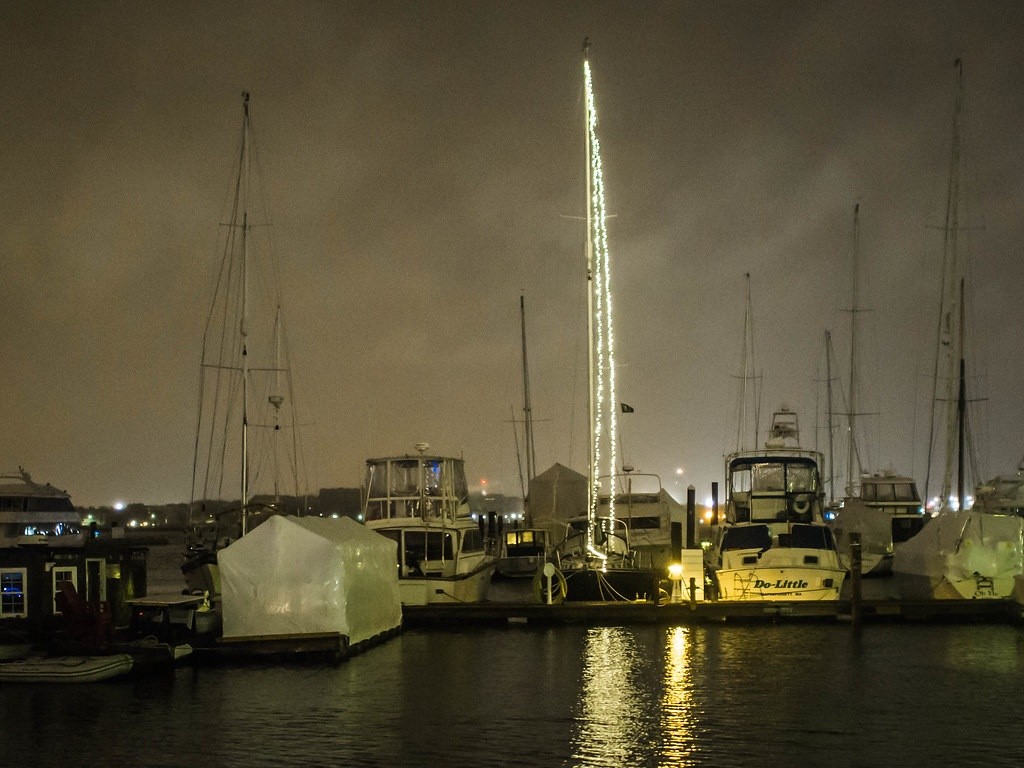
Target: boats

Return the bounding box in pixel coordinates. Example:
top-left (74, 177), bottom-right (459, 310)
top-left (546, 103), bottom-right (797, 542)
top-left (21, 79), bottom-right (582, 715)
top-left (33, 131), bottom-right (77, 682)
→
top-left (0, 465), bottom-right (86, 552)
top-left (0, 652), bottom-right (134, 683)
top-left (357, 442), bottom-right (499, 604)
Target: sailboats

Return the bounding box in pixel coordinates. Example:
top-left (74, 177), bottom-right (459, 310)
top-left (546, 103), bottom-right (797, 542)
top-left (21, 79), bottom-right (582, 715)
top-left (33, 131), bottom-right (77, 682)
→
top-left (543, 34), bottom-right (674, 601)
top-left (715, 272), bottom-right (848, 601)
top-left (181, 32), bottom-right (1023, 602)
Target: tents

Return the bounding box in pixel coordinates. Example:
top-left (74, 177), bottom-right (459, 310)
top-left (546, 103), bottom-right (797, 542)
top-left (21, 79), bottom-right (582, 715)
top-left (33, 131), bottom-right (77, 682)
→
top-left (219, 515), bottom-right (404, 643)
top-left (528, 463), bottom-right (587, 524)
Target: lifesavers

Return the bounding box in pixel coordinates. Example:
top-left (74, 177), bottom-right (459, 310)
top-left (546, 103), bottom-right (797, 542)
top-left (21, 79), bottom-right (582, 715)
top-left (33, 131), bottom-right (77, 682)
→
top-left (533, 564), bottom-right (568, 605)
top-left (792, 496), bottom-right (810, 513)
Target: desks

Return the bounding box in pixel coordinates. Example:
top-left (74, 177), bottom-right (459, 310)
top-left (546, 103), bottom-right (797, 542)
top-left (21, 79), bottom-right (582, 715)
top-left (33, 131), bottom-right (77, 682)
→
top-left (122, 595), bottom-right (207, 640)
